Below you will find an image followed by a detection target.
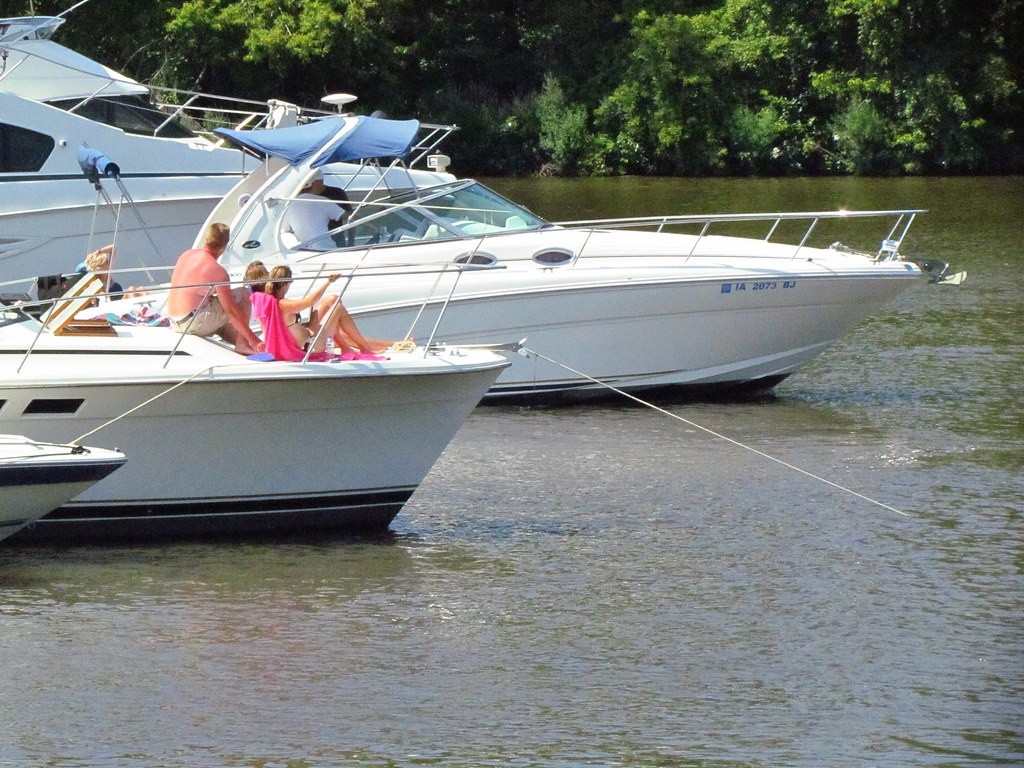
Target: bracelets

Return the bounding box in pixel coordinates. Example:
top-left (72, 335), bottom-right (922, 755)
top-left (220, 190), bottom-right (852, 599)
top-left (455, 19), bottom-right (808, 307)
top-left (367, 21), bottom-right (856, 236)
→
top-left (96, 248), bottom-right (102, 254)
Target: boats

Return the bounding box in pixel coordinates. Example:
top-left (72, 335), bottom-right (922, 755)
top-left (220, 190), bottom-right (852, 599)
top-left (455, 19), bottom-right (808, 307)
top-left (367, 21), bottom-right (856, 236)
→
top-left (1, 192), bottom-right (527, 542)
top-left (1, 1), bottom-right (967, 399)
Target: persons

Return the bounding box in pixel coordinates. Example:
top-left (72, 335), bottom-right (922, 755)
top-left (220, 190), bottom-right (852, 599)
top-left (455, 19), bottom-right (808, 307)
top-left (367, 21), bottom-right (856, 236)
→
top-left (168, 223), bottom-right (266, 354)
top-left (309, 170), bottom-right (356, 247)
top-left (265, 265), bottom-right (388, 361)
top-left (86, 245), bottom-right (150, 300)
top-left (244, 260), bottom-right (414, 353)
top-left (280, 179), bottom-right (378, 249)
